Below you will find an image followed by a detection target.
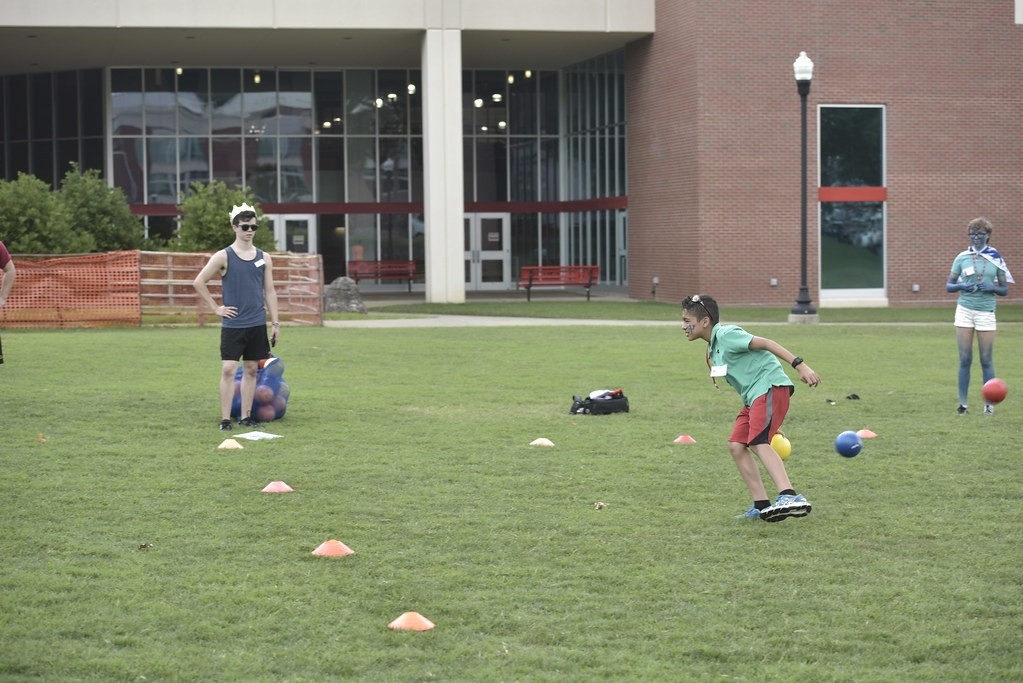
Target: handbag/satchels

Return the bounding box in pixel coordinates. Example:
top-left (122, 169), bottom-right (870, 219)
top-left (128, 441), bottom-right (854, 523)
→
top-left (570, 389), bottom-right (630, 414)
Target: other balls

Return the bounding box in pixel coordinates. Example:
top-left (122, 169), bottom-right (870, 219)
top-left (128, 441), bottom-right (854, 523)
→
top-left (231, 354), bottom-right (290, 422)
top-left (834, 431), bottom-right (862, 458)
top-left (982, 378), bottom-right (1008, 404)
top-left (770, 434), bottom-right (791, 461)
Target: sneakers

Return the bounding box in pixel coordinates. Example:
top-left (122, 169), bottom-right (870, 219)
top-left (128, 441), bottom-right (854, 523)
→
top-left (759, 493), bottom-right (812, 523)
top-left (733, 504), bottom-right (761, 520)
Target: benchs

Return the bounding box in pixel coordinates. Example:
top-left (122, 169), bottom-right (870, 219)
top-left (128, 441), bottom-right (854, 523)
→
top-left (347, 261), bottom-right (416, 293)
top-left (519, 265), bottom-right (600, 304)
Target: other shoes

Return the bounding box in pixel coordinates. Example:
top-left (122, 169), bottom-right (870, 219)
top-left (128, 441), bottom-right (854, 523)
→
top-left (984, 404), bottom-right (994, 416)
top-left (238, 417), bottom-right (265, 431)
top-left (220, 419), bottom-right (232, 431)
top-left (952, 404), bottom-right (969, 416)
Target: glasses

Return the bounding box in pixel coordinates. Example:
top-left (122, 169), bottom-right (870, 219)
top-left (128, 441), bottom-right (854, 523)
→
top-left (967, 232), bottom-right (988, 239)
top-left (690, 294), bottom-right (713, 321)
top-left (238, 224), bottom-right (259, 231)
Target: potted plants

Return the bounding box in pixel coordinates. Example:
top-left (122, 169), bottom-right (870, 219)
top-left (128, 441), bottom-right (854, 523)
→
top-left (139, 178), bottom-right (325, 328)
top-left (0, 160), bottom-right (141, 329)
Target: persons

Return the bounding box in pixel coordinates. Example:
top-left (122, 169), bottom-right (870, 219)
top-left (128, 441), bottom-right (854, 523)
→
top-left (946, 216), bottom-right (1008, 416)
top-left (192, 202), bottom-right (280, 431)
top-left (0, 241), bottom-right (17, 366)
top-left (682, 294), bottom-right (822, 521)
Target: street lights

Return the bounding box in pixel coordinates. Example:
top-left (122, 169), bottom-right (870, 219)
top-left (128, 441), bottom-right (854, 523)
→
top-left (384, 158), bottom-right (394, 261)
top-left (791, 50), bottom-right (818, 315)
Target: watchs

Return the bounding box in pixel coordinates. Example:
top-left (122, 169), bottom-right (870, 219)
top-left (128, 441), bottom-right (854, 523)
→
top-left (791, 355), bottom-right (803, 367)
top-left (271, 321), bottom-right (280, 327)
